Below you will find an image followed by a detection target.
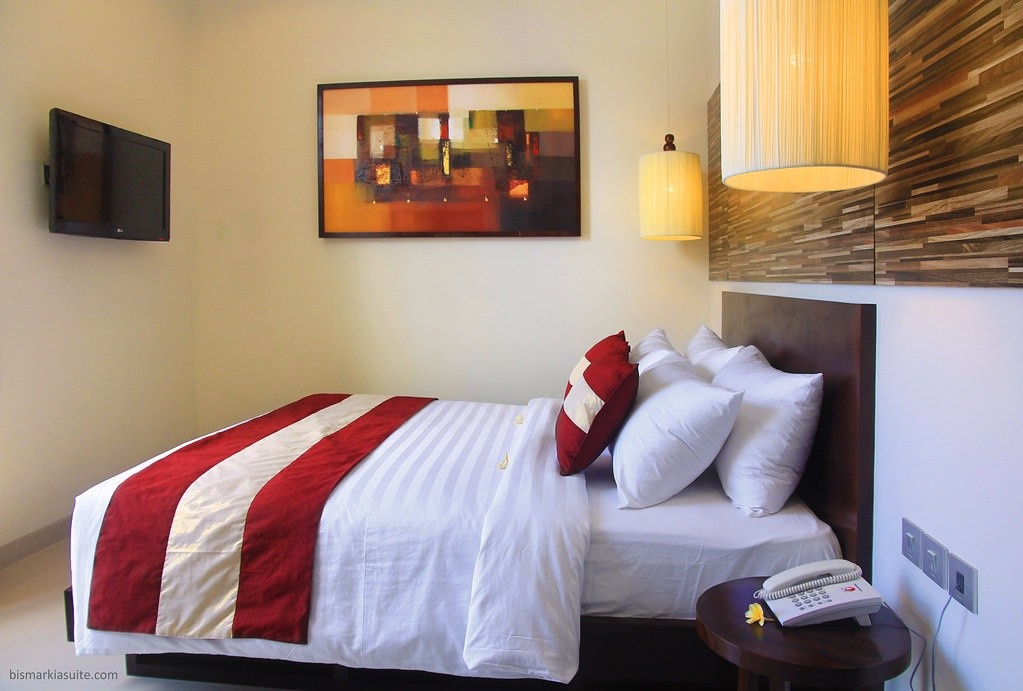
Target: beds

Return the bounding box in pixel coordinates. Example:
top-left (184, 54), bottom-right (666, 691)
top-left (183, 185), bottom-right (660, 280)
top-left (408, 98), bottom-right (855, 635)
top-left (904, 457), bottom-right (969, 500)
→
top-left (64, 290), bottom-right (878, 691)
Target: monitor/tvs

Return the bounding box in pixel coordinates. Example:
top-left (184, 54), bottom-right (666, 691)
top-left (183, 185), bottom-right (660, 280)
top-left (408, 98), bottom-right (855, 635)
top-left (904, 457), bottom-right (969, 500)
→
top-left (47, 107), bottom-right (171, 242)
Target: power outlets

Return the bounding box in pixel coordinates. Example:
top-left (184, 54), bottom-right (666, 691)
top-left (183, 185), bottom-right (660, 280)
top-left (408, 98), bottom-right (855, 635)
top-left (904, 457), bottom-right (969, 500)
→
top-left (922, 534), bottom-right (948, 590)
top-left (902, 517), bottom-right (923, 570)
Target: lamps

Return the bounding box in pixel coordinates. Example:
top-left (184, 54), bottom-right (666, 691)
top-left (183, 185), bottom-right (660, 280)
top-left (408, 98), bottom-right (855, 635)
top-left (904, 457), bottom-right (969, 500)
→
top-left (638, 0), bottom-right (703, 241)
top-left (720, 0), bottom-right (889, 192)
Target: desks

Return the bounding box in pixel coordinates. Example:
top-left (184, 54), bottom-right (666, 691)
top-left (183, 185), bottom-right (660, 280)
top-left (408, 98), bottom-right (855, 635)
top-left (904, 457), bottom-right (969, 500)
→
top-left (695, 575), bottom-right (912, 691)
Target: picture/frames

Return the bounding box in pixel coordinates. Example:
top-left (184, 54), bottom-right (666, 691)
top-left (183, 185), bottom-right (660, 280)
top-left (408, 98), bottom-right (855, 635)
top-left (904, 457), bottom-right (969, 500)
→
top-left (317, 77), bottom-right (581, 238)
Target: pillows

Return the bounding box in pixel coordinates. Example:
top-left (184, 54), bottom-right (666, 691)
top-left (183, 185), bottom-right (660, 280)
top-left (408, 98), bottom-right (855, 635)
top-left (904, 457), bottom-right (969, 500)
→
top-left (554, 329), bottom-right (824, 517)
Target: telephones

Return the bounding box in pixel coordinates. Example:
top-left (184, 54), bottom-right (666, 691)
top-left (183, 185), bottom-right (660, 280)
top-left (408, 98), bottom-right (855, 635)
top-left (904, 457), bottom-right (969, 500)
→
top-left (758, 558), bottom-right (883, 628)
